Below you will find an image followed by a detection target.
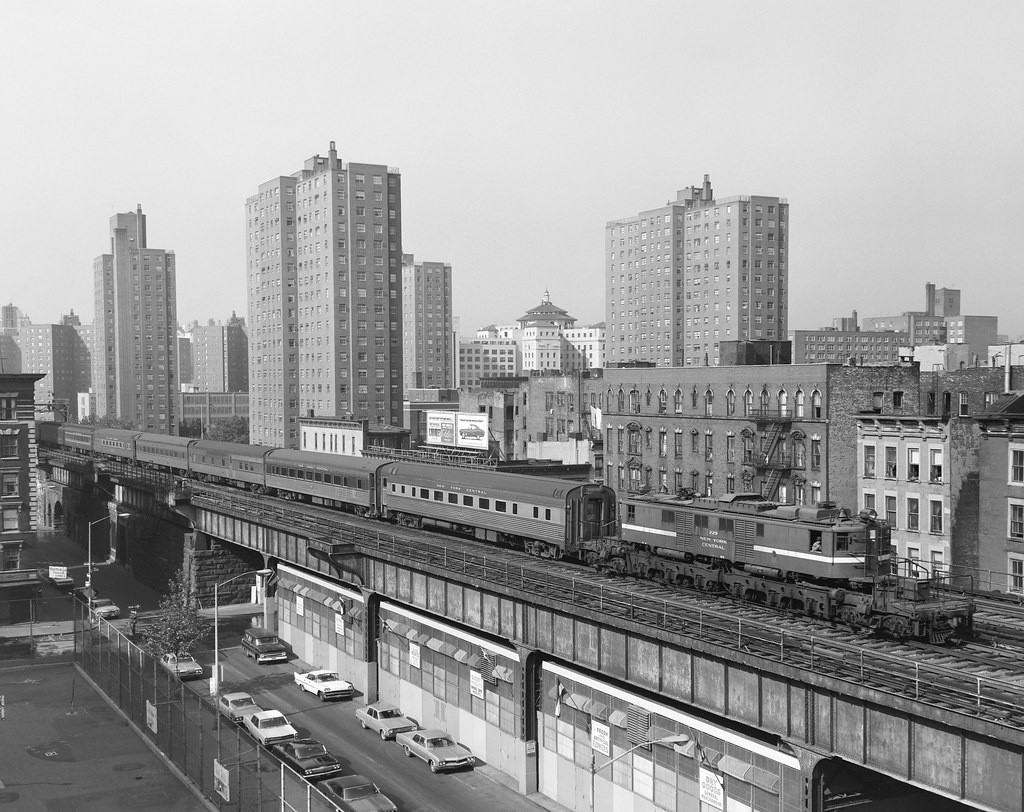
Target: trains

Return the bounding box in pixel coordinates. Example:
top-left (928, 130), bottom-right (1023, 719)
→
top-left (35, 421), bottom-right (978, 647)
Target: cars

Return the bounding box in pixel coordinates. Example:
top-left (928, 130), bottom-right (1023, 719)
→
top-left (356, 701), bottom-right (418, 741)
top-left (91, 596), bottom-right (121, 620)
top-left (314, 773), bottom-right (398, 812)
top-left (459, 423), bottom-right (485, 440)
top-left (72, 586), bottom-right (98, 603)
top-left (293, 667), bottom-right (355, 703)
top-left (241, 708), bottom-right (299, 746)
top-left (270, 735), bottom-right (343, 779)
top-left (395, 729), bottom-right (476, 773)
top-left (161, 650), bottom-right (204, 681)
top-left (216, 692), bottom-right (263, 726)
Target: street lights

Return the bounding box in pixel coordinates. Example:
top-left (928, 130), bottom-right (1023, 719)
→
top-left (88, 512), bottom-right (131, 625)
top-left (212, 568), bottom-right (272, 695)
top-left (591, 733), bottom-right (690, 812)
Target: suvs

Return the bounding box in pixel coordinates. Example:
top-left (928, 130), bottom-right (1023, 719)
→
top-left (242, 627), bottom-right (288, 665)
